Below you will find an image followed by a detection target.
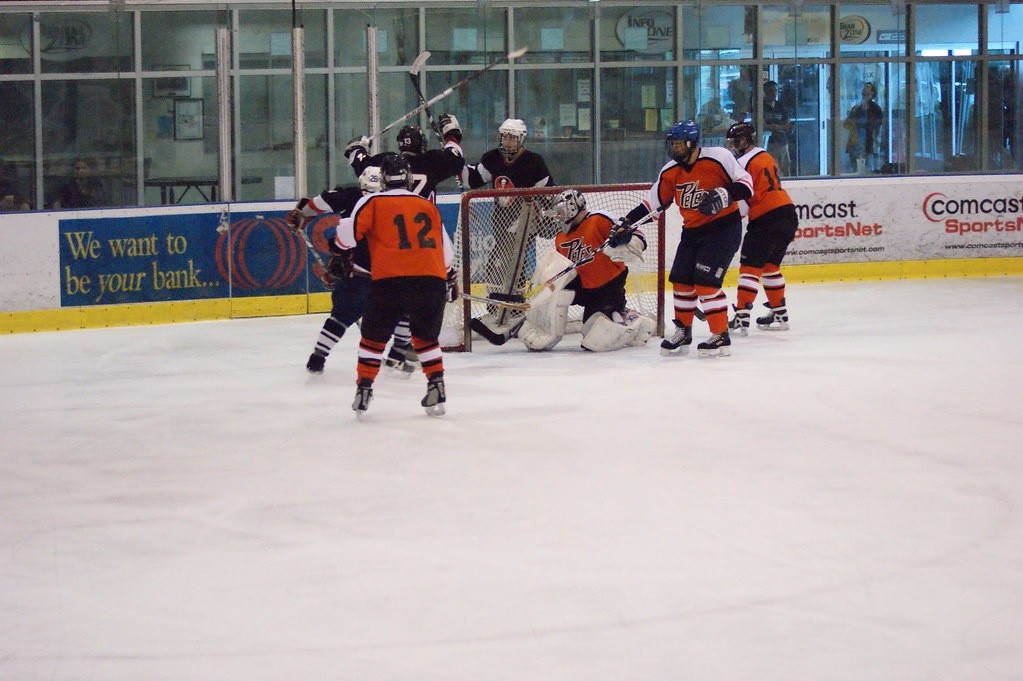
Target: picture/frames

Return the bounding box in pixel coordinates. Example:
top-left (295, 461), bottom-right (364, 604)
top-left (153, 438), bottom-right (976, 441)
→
top-left (173, 98), bottom-right (204, 139)
top-left (153, 64), bottom-right (192, 96)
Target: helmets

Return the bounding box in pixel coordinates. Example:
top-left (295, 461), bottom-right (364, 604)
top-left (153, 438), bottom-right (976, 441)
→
top-left (498, 118), bottom-right (527, 150)
top-left (380, 155), bottom-right (410, 188)
top-left (552, 189), bottom-right (586, 232)
top-left (726, 122), bottom-right (756, 155)
top-left (359, 165), bottom-right (386, 195)
top-left (397, 125), bottom-right (423, 152)
top-left (666, 121), bottom-right (697, 159)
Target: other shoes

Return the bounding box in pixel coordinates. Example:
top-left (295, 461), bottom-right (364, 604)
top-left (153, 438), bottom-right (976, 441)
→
top-left (405, 347), bottom-right (419, 364)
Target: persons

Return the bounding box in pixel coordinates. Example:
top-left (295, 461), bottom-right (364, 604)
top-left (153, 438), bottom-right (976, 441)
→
top-left (608, 120), bottom-right (755, 350)
top-left (0, 146), bottom-right (30, 212)
top-left (51, 157), bottom-right (112, 208)
top-left (334, 154), bottom-right (455, 410)
top-left (763, 82), bottom-right (795, 177)
top-left (518, 191), bottom-right (647, 352)
top-left (285, 165), bottom-right (420, 374)
top-left (345, 113), bottom-right (465, 352)
top-left (726, 122), bottom-right (798, 329)
top-left (455, 118), bottom-right (558, 315)
top-left (843, 82), bottom-right (883, 174)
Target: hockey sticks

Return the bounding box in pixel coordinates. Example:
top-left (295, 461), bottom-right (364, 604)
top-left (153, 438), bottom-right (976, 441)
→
top-left (409, 50), bottom-right (447, 149)
top-left (299, 227), bottom-right (413, 374)
top-left (353, 269), bottom-right (557, 310)
top-left (361, 48), bottom-right (528, 141)
top-left (468, 313), bottom-right (528, 346)
top-left (486, 197), bottom-right (676, 304)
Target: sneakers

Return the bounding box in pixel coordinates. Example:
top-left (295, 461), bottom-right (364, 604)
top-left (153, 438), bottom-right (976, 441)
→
top-left (351, 386), bottom-right (373, 417)
top-left (728, 302), bottom-right (752, 337)
top-left (660, 319), bottom-right (692, 354)
top-left (383, 357), bottom-right (416, 379)
top-left (305, 352), bottom-right (327, 377)
top-left (756, 298), bottom-right (791, 330)
top-left (421, 380), bottom-right (446, 414)
top-left (696, 331), bottom-right (732, 359)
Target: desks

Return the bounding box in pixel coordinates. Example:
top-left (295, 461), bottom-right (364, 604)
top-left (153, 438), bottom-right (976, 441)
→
top-left (144, 176), bottom-right (263, 205)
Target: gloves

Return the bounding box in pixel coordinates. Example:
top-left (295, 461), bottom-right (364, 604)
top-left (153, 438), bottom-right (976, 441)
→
top-left (287, 198), bottom-right (314, 236)
top-left (447, 269), bottom-right (459, 303)
top-left (322, 258), bottom-right (345, 290)
top-left (699, 187), bottom-right (729, 216)
top-left (438, 112), bottom-right (463, 141)
top-left (344, 134), bottom-right (372, 158)
top-left (608, 218), bottom-right (633, 247)
